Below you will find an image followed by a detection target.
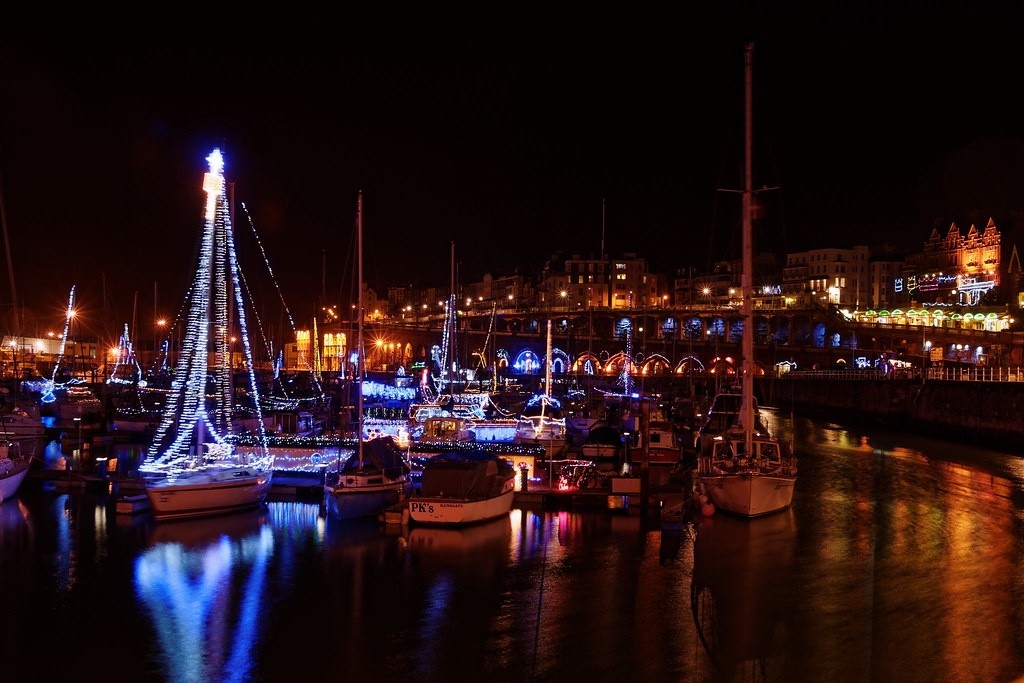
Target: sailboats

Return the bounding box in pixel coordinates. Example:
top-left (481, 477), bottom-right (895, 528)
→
top-left (0, 41), bottom-right (803, 531)
